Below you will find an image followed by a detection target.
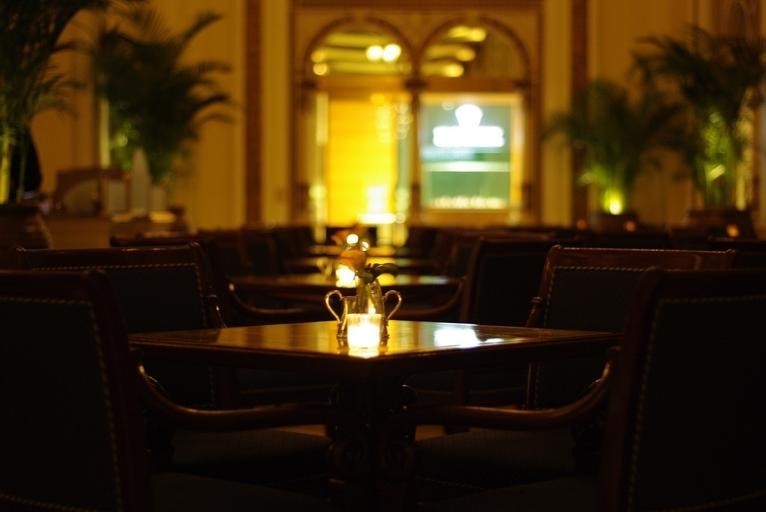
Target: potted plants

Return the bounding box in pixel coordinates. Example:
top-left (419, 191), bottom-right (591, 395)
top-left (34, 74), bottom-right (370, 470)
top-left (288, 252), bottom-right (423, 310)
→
top-left (627, 23), bottom-right (766, 232)
top-left (550, 73), bottom-right (664, 232)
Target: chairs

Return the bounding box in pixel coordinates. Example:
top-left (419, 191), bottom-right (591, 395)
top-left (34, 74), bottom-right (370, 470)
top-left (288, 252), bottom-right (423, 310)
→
top-left (0, 226), bottom-right (765, 511)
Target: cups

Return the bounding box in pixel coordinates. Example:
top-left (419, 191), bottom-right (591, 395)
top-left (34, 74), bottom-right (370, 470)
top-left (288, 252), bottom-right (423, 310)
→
top-left (323, 287), bottom-right (406, 350)
